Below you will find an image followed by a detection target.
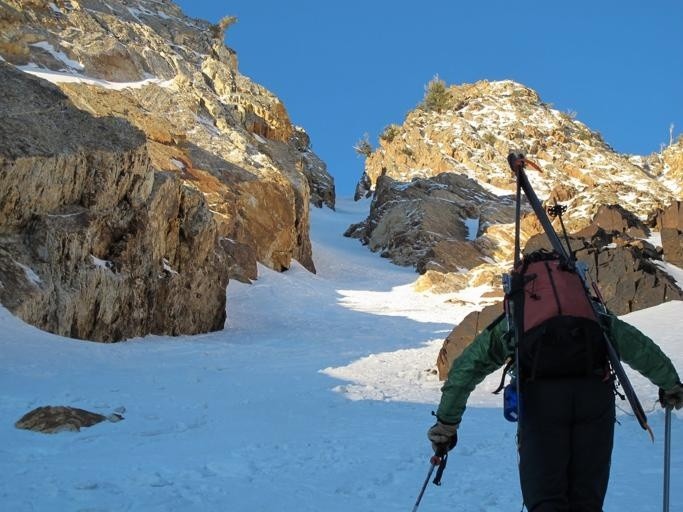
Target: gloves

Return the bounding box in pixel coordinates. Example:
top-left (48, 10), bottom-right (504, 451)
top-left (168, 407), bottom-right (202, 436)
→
top-left (427, 420), bottom-right (456, 452)
top-left (658, 382), bottom-right (682, 410)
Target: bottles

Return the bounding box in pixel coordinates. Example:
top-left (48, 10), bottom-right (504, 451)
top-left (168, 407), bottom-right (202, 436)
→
top-left (502, 378), bottom-right (517, 424)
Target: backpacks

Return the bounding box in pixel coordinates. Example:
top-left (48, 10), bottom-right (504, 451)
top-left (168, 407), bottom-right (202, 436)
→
top-left (504, 248), bottom-right (611, 379)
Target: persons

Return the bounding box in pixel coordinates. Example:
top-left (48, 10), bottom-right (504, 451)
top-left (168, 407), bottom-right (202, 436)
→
top-left (429, 299), bottom-right (683, 512)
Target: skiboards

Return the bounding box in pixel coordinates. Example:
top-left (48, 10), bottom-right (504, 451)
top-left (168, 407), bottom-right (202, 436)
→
top-left (499, 151), bottom-right (648, 441)
